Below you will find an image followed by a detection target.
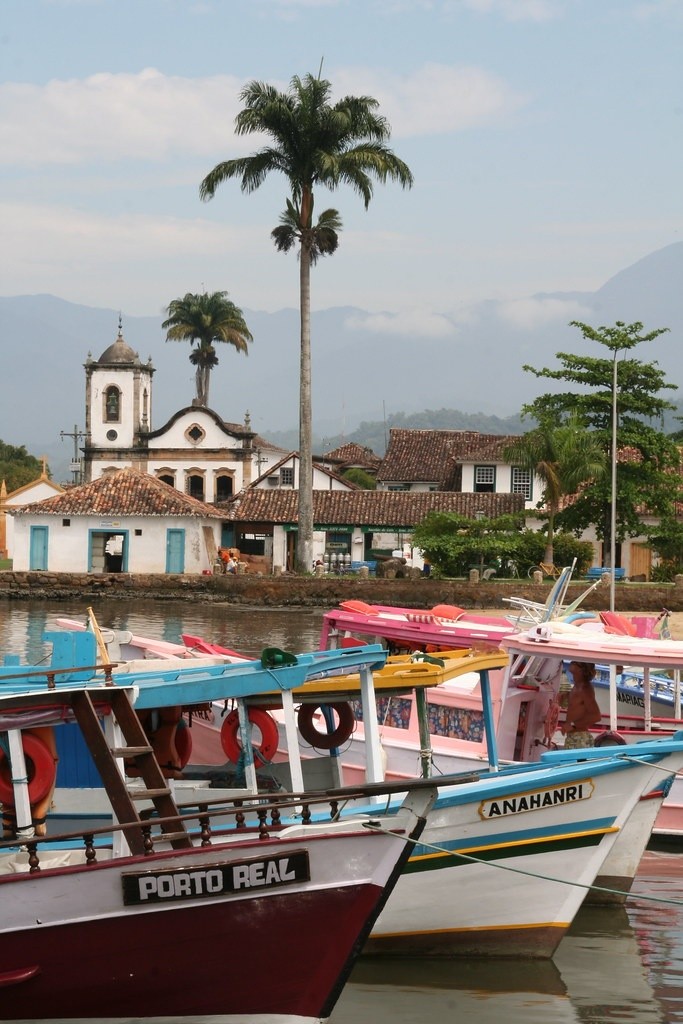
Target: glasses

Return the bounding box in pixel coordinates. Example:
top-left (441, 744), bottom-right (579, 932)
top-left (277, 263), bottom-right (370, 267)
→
top-left (570, 661), bottom-right (583, 669)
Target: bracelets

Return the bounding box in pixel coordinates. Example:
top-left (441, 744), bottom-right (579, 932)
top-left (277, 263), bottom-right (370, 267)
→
top-left (571, 722), bottom-right (577, 730)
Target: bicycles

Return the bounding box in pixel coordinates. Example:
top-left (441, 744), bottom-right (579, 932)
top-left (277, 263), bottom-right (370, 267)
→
top-left (527, 559), bottom-right (564, 582)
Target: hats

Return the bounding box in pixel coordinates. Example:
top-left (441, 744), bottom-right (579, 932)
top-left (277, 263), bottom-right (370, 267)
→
top-left (233, 557), bottom-right (237, 559)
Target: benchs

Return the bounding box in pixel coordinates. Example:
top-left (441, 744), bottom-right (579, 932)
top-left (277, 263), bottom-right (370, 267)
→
top-left (584, 567), bottom-right (626, 583)
top-left (347, 561), bottom-right (377, 575)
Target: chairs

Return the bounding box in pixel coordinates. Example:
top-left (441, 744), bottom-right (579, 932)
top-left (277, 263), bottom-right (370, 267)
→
top-left (502, 557), bottom-right (602, 628)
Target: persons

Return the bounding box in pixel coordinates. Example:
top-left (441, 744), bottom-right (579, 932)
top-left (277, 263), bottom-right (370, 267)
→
top-left (315, 560), bottom-right (324, 574)
top-left (227, 553), bottom-right (238, 574)
top-left (557, 661), bottom-right (601, 749)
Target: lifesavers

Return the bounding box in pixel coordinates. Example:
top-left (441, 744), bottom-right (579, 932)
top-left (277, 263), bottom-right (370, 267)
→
top-left (157, 718), bottom-right (194, 768)
top-left (594, 732), bottom-right (628, 747)
top-left (223, 709), bottom-right (278, 766)
top-left (300, 700), bottom-right (354, 747)
top-left (0, 731), bottom-right (57, 808)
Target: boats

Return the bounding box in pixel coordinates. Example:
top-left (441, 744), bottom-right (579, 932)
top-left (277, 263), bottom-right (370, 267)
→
top-left (0, 666), bottom-right (478, 1024)
top-left (53, 613), bottom-right (683, 836)
top-left (179, 600), bottom-right (683, 742)
top-left (0, 632), bottom-right (683, 962)
